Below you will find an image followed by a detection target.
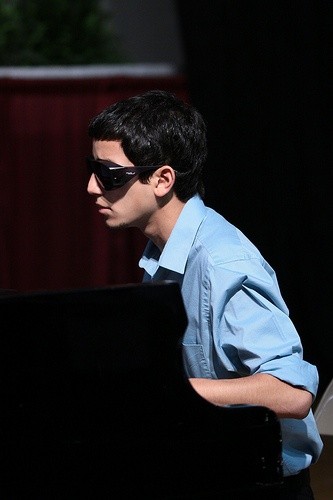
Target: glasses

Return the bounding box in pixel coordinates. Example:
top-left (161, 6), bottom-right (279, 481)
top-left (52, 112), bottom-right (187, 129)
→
top-left (87, 157), bottom-right (162, 192)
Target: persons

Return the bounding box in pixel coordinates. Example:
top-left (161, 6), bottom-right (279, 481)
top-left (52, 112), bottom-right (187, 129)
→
top-left (84, 91), bottom-right (324, 500)
